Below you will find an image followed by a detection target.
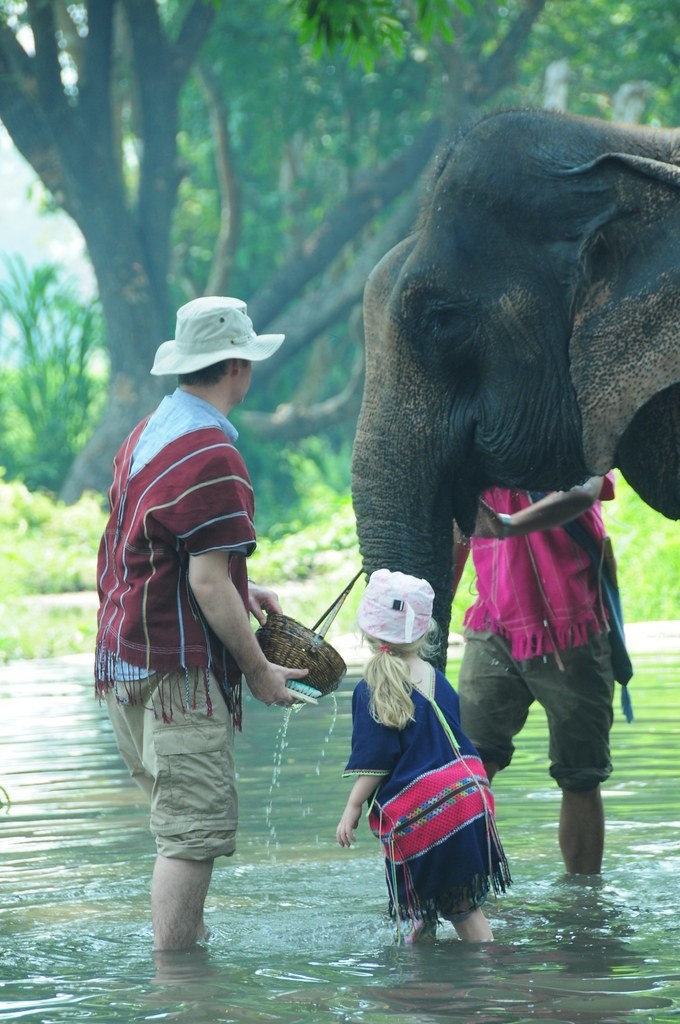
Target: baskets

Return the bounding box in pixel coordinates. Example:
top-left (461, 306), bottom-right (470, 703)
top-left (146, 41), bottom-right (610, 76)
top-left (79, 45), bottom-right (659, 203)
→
top-left (255, 569), bottom-right (364, 698)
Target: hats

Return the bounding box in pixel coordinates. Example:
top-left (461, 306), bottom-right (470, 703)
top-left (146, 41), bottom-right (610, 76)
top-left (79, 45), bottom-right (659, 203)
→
top-left (357, 568), bottom-right (433, 644)
top-left (150, 296), bottom-right (286, 376)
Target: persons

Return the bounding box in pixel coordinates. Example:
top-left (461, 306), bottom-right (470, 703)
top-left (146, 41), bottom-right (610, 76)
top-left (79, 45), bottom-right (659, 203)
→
top-left (95, 294), bottom-right (310, 952)
top-left (336, 569), bottom-right (514, 944)
top-left (448, 470), bottom-right (616, 872)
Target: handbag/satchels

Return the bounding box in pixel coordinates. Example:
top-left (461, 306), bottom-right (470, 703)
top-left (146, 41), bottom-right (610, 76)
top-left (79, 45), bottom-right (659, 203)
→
top-left (586, 536), bottom-right (634, 727)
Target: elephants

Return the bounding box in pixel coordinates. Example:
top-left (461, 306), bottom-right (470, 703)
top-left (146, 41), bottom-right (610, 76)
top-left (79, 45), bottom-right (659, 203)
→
top-left (349, 102), bottom-right (680, 675)
